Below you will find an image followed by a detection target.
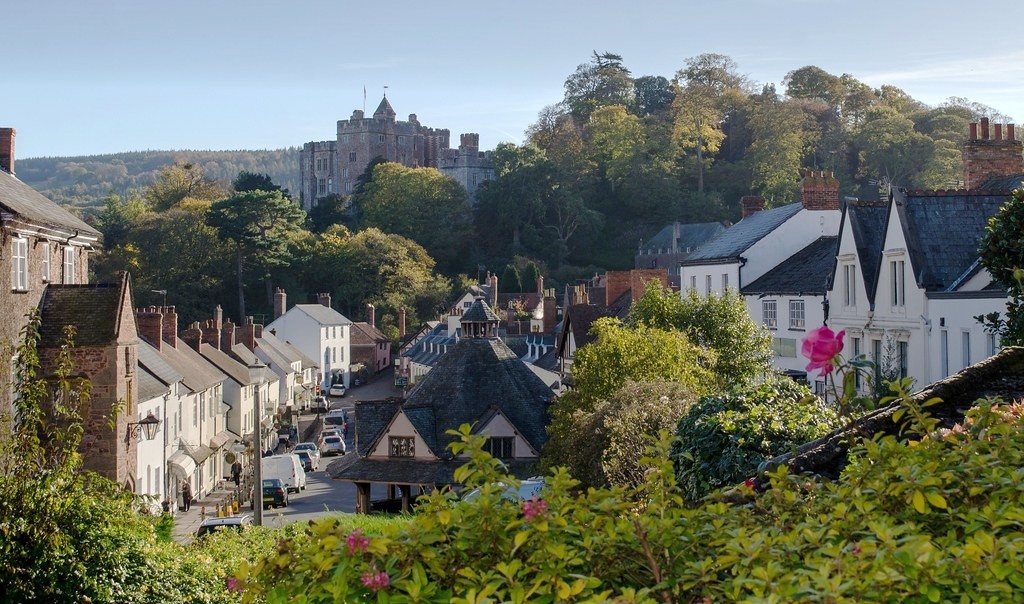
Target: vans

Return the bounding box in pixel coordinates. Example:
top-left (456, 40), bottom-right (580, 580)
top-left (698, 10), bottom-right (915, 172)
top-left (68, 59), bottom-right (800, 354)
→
top-left (260, 454), bottom-right (306, 493)
top-left (322, 415), bottom-right (347, 433)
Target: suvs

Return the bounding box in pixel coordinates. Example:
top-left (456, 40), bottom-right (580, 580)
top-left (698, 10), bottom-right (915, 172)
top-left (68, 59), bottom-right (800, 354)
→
top-left (311, 397), bottom-right (331, 412)
top-left (291, 448), bottom-right (317, 471)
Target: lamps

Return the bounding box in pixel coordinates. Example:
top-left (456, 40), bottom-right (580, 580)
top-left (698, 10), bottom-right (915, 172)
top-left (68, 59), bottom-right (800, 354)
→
top-left (128, 411), bottom-right (160, 447)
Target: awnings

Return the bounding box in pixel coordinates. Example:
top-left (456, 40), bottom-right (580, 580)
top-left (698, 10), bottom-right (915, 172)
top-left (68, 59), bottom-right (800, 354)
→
top-left (171, 453), bottom-right (196, 479)
top-left (210, 432), bottom-right (230, 448)
top-left (192, 444), bottom-right (215, 466)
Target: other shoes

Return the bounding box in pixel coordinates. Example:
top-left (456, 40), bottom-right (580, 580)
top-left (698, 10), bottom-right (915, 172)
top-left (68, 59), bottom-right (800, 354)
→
top-left (186, 510), bottom-right (189, 512)
top-left (182, 510), bottom-right (186, 511)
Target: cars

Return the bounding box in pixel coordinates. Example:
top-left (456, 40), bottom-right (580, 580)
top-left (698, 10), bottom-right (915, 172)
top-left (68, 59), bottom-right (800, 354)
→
top-left (250, 478), bottom-right (289, 509)
top-left (330, 383), bottom-right (346, 397)
top-left (318, 428), bottom-right (345, 443)
top-left (319, 437), bottom-right (346, 455)
top-left (191, 515), bottom-right (255, 544)
top-left (296, 444), bottom-right (321, 462)
top-left (330, 409), bottom-right (348, 426)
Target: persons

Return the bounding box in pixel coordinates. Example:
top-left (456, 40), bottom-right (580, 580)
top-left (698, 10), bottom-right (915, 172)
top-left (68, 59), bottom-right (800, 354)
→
top-left (285, 436), bottom-right (290, 449)
top-left (180, 479), bottom-right (191, 512)
top-left (159, 495), bottom-right (171, 512)
top-left (230, 460), bottom-right (242, 487)
top-left (261, 449), bottom-right (266, 458)
top-left (266, 447), bottom-right (273, 456)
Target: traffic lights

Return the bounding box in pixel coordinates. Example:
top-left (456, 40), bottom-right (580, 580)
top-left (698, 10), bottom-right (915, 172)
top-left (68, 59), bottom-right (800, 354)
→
top-left (316, 386), bottom-right (319, 394)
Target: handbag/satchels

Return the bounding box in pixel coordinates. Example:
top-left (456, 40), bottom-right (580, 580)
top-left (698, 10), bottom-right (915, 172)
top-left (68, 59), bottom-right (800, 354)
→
top-left (229, 473), bottom-right (235, 482)
top-left (188, 495), bottom-right (193, 500)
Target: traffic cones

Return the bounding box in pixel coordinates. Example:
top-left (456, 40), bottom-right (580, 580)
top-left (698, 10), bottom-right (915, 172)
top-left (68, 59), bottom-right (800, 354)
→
top-left (218, 510), bottom-right (224, 518)
top-left (232, 501), bottom-right (239, 514)
top-left (226, 505), bottom-right (234, 517)
